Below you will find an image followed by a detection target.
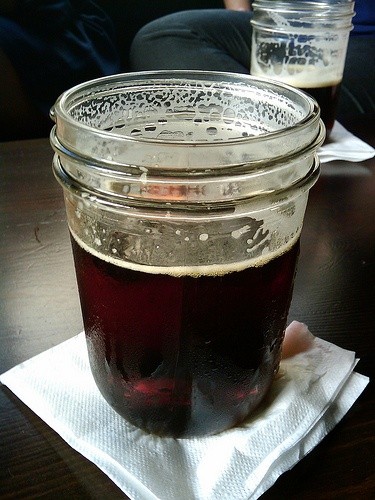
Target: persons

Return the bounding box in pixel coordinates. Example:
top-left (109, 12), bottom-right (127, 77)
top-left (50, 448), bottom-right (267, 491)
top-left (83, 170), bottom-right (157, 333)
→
top-left (130, 0), bottom-right (375, 134)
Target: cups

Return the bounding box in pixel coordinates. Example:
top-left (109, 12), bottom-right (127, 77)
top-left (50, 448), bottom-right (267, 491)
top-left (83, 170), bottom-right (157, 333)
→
top-left (249, 0), bottom-right (356, 146)
top-left (49, 69), bottom-right (327, 439)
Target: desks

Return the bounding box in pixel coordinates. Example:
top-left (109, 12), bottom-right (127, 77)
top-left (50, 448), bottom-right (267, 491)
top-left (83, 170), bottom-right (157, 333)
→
top-left (0, 138), bottom-right (375, 500)
